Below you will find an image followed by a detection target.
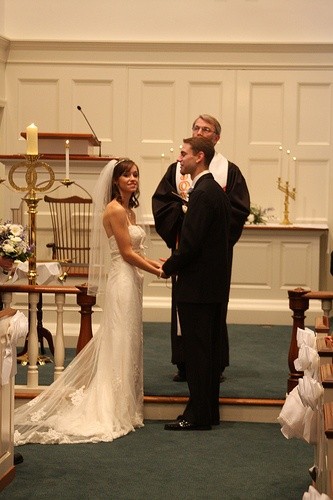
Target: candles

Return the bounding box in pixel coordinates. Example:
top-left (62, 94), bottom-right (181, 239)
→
top-left (170, 149), bottom-right (174, 165)
top-left (160, 153), bottom-right (165, 176)
top-left (66, 140), bottom-right (70, 181)
top-left (278, 146), bottom-right (296, 189)
top-left (26, 123), bottom-right (39, 155)
top-left (179, 145), bottom-right (183, 155)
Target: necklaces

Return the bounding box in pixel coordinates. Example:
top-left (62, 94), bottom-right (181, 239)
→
top-left (129, 213), bottom-right (131, 218)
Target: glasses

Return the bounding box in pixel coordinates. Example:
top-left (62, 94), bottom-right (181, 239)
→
top-left (192, 126), bottom-right (216, 134)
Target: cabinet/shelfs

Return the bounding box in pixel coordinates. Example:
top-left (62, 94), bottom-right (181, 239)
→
top-left (288, 286), bottom-right (329, 396)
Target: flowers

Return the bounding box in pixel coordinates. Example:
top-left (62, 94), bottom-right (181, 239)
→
top-left (0, 219), bottom-right (32, 274)
top-left (246, 214), bottom-right (255, 225)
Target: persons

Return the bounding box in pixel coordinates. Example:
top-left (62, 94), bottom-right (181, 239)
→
top-left (160, 135), bottom-right (232, 430)
top-left (14, 159), bottom-right (164, 443)
top-left (152, 115), bottom-right (250, 383)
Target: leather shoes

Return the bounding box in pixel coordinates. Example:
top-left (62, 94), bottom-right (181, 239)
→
top-left (178, 414), bottom-right (185, 420)
top-left (164, 423), bottom-right (212, 431)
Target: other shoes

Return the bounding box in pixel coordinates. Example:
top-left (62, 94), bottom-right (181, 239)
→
top-left (174, 373), bottom-right (187, 381)
top-left (219, 376), bottom-right (224, 382)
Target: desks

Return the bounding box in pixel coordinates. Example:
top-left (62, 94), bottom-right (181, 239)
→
top-left (15, 262), bottom-right (63, 364)
top-left (136, 224), bottom-right (329, 326)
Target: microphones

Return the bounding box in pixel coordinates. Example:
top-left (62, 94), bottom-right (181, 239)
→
top-left (77, 106), bottom-right (97, 139)
